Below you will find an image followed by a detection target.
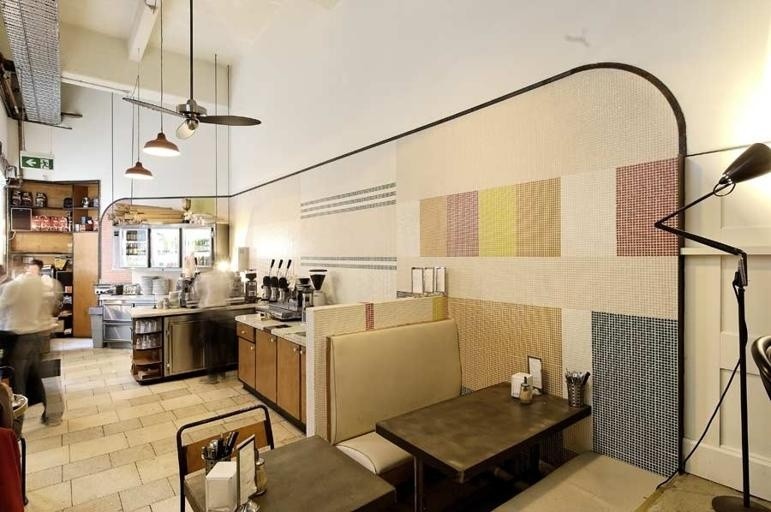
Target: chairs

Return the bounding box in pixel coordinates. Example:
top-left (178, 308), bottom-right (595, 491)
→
top-left (177, 404), bottom-right (275, 512)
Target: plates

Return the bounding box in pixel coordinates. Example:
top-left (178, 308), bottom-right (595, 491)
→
top-left (140, 276), bottom-right (170, 295)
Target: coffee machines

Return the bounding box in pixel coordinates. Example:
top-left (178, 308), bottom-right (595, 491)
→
top-left (294, 279), bottom-right (311, 311)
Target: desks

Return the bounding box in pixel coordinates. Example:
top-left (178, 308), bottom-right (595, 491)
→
top-left (377, 381), bottom-right (593, 499)
top-left (181, 434), bottom-right (397, 512)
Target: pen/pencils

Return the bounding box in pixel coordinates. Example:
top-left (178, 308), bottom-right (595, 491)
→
top-left (201, 430), bottom-right (239, 460)
top-left (565, 368), bottom-right (590, 384)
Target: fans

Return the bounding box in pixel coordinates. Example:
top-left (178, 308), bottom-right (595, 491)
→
top-left (123, 0), bottom-right (267, 135)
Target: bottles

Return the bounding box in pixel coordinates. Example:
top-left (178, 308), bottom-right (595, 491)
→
top-left (519, 383), bottom-right (533, 404)
top-left (135, 320), bottom-right (160, 348)
top-left (128, 232), bottom-right (146, 255)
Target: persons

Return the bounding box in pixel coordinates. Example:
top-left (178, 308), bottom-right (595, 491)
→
top-left (0, 260), bottom-right (64, 428)
top-left (192, 265), bottom-right (233, 386)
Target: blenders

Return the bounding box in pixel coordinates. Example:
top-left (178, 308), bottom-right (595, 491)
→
top-left (310, 269), bottom-right (326, 307)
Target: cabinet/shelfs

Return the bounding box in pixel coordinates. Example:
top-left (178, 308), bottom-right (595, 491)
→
top-left (131, 315), bottom-right (164, 383)
top-left (6, 175), bottom-right (101, 338)
top-left (236, 320), bottom-right (307, 426)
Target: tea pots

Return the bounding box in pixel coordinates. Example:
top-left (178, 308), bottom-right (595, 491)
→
top-left (261, 278), bottom-right (288, 301)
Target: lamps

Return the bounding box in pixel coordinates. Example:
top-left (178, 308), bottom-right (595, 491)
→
top-left (655, 142), bottom-right (770, 511)
top-left (126, 1), bottom-right (178, 181)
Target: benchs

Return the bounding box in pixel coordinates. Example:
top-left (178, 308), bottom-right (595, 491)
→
top-left (487, 452), bottom-right (666, 511)
top-left (327, 317), bottom-right (463, 489)
